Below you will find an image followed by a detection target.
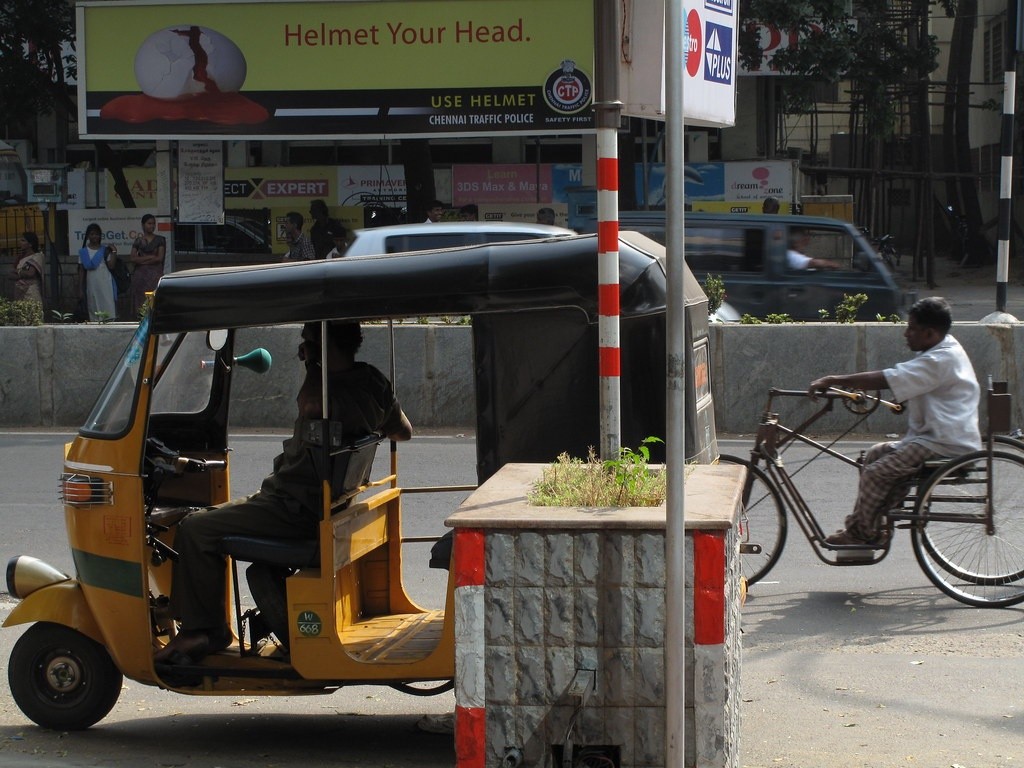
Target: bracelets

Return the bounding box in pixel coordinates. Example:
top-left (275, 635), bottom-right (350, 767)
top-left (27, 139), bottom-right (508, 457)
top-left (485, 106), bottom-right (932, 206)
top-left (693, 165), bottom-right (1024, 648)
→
top-left (112, 251), bottom-right (116, 252)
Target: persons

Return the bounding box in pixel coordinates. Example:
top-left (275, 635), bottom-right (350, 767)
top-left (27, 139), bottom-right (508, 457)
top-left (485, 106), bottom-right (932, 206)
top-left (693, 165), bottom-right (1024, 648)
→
top-left (8, 231), bottom-right (45, 311)
top-left (761, 197), bottom-right (780, 214)
top-left (536, 208), bottom-right (555, 226)
top-left (282, 200), bottom-right (349, 261)
top-left (79, 223), bottom-right (119, 320)
top-left (787, 232), bottom-right (841, 269)
top-left (130, 214), bottom-right (167, 310)
top-left (423, 200), bottom-right (444, 223)
top-left (809, 297), bottom-right (983, 546)
top-left (154, 319), bottom-right (413, 664)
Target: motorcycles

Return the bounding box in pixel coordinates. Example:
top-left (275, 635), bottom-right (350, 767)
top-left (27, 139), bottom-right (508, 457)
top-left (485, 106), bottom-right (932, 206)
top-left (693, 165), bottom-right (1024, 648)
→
top-left (2, 231), bottom-right (721, 734)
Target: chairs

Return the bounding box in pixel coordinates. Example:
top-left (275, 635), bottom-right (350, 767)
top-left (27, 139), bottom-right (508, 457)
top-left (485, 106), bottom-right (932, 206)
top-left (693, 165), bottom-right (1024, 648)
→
top-left (216, 427), bottom-right (387, 659)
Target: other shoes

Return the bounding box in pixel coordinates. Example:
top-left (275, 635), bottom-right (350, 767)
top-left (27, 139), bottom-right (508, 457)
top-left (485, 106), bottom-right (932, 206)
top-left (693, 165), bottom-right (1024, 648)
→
top-left (826, 527), bottom-right (882, 544)
top-left (154, 623), bottom-right (234, 665)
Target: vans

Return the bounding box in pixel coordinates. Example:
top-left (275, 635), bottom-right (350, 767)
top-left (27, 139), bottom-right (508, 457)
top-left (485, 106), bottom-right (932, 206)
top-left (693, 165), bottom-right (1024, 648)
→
top-left (569, 213), bottom-right (908, 324)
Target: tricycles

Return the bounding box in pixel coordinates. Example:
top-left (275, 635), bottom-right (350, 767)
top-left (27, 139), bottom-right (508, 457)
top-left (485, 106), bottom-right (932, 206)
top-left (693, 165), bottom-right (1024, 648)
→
top-left (717, 382), bottom-right (1024, 610)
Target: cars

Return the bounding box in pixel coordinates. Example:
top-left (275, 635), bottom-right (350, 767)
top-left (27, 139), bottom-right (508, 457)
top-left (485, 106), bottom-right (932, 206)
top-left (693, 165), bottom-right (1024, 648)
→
top-left (338, 216), bottom-right (584, 263)
top-left (170, 217), bottom-right (275, 252)
